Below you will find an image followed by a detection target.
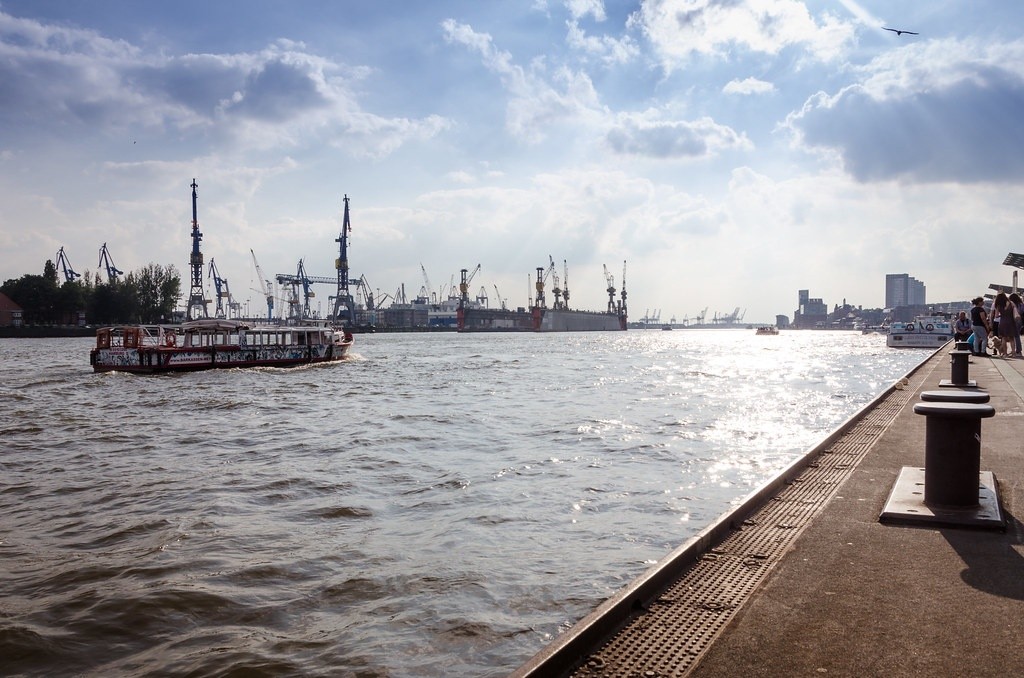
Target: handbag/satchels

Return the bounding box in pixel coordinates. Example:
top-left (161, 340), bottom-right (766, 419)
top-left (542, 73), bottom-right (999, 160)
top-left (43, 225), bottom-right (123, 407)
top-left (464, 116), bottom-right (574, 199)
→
top-left (1010, 301), bottom-right (1021, 322)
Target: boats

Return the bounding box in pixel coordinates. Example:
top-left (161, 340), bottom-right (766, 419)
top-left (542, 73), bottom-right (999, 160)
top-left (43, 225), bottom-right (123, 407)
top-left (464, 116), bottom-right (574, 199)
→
top-left (662, 322), bottom-right (672, 331)
top-left (755, 324), bottom-right (779, 336)
top-left (89, 319), bottom-right (352, 370)
top-left (886, 307), bottom-right (955, 347)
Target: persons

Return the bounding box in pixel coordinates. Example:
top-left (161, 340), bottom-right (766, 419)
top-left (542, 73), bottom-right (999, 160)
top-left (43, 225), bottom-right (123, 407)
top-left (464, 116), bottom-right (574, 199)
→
top-left (954, 292), bottom-right (1024, 359)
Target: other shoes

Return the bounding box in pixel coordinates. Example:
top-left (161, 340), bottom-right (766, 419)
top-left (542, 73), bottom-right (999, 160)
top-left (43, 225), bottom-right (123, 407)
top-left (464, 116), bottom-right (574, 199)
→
top-left (972, 350), bottom-right (979, 356)
top-left (979, 351), bottom-right (992, 357)
top-left (1011, 353), bottom-right (1016, 357)
top-left (1002, 353), bottom-right (1008, 359)
top-left (1015, 352), bottom-right (1022, 356)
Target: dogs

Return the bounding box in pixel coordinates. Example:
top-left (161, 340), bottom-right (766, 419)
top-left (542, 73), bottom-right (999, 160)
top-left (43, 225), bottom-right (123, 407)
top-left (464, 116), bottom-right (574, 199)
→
top-left (990, 336), bottom-right (1007, 359)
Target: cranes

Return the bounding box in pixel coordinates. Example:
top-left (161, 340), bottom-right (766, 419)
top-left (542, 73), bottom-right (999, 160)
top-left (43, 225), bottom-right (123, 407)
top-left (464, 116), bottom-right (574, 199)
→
top-left (55, 246), bottom-right (81, 282)
top-left (333, 192), bottom-right (352, 332)
top-left (207, 247), bottom-right (628, 333)
top-left (185, 178), bottom-right (212, 322)
top-left (97, 241), bottom-right (124, 286)
top-left (639, 306), bottom-right (747, 327)
